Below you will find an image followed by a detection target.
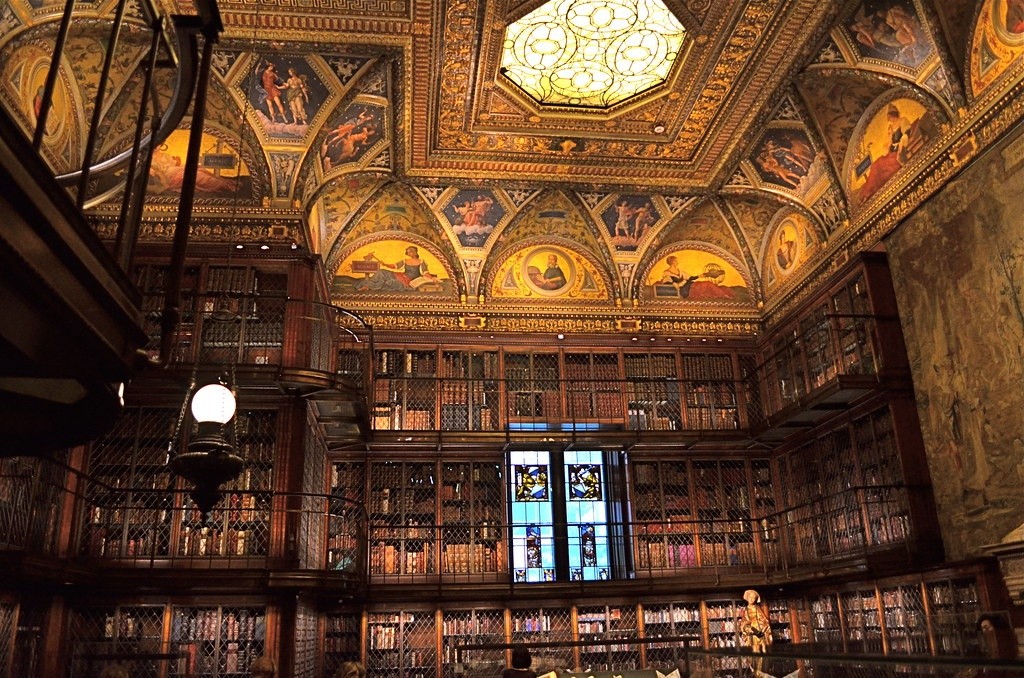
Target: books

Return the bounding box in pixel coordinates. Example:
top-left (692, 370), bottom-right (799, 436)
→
top-left (0, 267), bottom-right (1024, 678)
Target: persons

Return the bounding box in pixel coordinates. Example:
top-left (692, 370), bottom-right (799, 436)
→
top-left (977, 614), bottom-right (1006, 631)
top-left (740, 590), bottom-right (773, 652)
top-left (503, 646), bottom-right (536, 678)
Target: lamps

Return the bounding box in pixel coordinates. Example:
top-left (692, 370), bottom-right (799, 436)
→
top-left (166, 0), bottom-right (261, 531)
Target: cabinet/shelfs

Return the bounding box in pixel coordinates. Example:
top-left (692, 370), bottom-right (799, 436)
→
top-left (0, 250), bottom-right (1024, 677)
